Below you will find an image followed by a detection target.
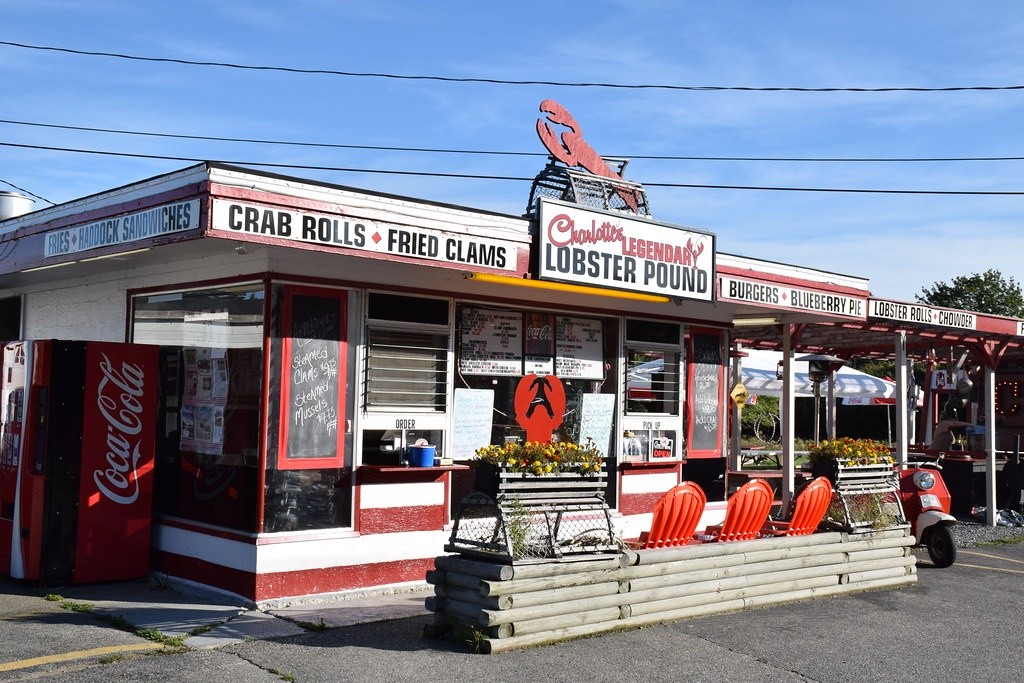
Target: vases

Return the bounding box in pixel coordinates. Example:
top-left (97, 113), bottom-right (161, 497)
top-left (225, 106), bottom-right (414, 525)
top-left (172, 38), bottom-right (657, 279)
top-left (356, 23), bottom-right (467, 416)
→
top-left (808, 457), bottom-right (895, 490)
top-left (471, 459), bottom-right (606, 501)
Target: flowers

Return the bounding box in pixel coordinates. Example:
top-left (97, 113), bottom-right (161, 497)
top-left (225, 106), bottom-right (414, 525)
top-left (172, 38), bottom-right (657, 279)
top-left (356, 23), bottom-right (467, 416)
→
top-left (475, 434), bottom-right (606, 478)
top-left (808, 434), bottom-right (897, 468)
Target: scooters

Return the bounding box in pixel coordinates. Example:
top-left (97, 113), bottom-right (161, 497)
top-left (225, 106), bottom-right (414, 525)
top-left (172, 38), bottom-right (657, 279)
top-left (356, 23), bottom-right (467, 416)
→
top-left (827, 451), bottom-right (957, 568)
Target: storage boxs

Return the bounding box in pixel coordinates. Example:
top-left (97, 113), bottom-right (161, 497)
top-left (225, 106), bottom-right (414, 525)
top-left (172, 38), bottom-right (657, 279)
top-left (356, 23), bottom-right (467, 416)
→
top-left (410, 444), bottom-right (436, 468)
top-left (433, 457), bottom-right (453, 467)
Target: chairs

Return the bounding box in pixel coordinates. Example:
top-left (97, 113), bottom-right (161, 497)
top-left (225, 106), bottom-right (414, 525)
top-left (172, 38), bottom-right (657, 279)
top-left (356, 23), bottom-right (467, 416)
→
top-left (620, 480), bottom-right (707, 551)
top-left (694, 476), bottom-right (775, 541)
top-left (754, 475), bottom-right (831, 538)
